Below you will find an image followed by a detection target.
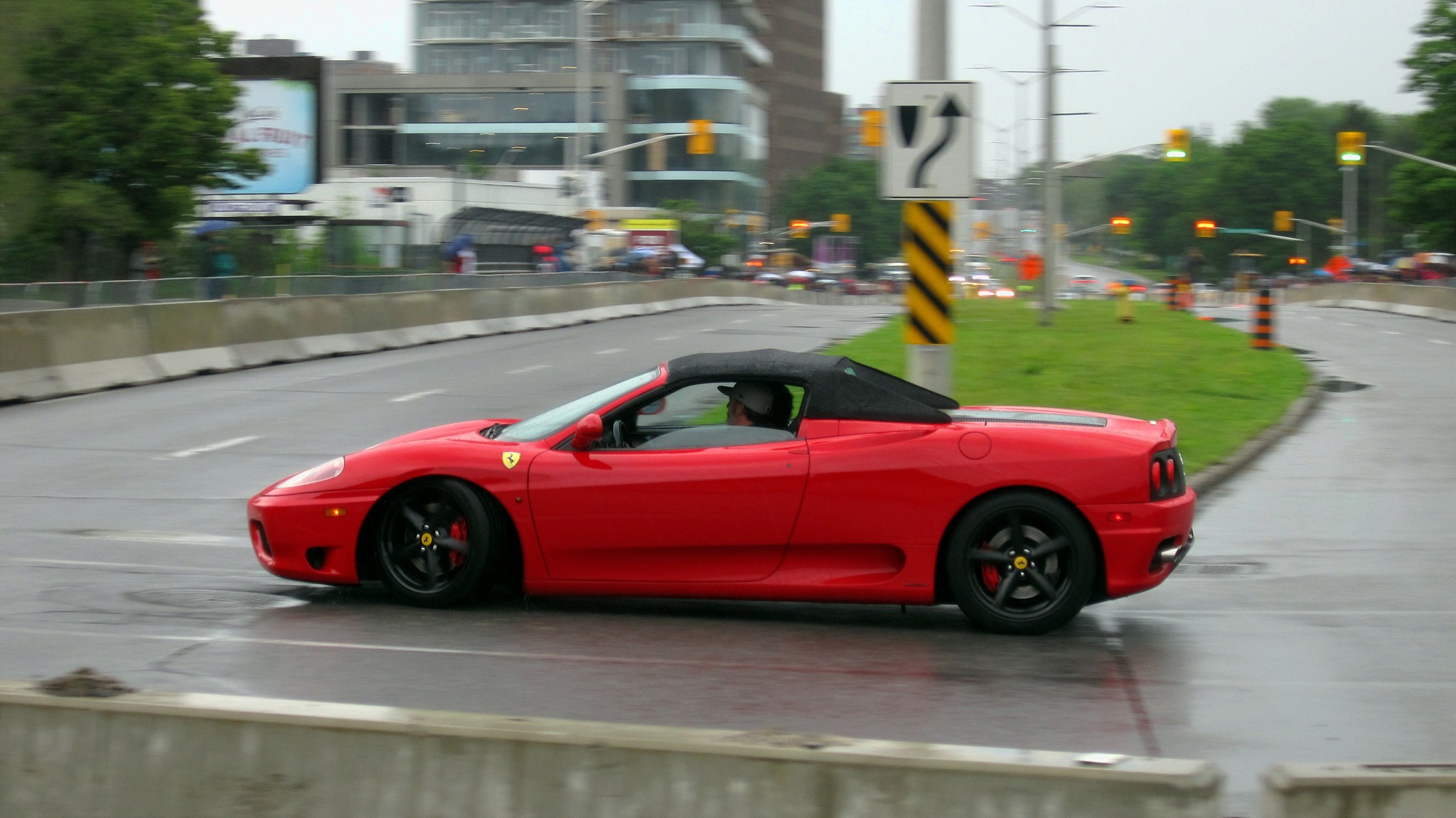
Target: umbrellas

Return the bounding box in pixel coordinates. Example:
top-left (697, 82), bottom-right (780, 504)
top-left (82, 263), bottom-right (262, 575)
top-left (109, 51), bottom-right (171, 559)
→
top-left (192, 222), bottom-right (243, 249)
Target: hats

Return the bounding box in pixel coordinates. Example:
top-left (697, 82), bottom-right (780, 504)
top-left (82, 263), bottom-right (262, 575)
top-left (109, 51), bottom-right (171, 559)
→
top-left (717, 377), bottom-right (775, 415)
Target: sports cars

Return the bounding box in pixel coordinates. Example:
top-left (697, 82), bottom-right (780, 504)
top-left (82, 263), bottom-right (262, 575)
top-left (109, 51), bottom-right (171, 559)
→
top-left (247, 347), bottom-right (1201, 637)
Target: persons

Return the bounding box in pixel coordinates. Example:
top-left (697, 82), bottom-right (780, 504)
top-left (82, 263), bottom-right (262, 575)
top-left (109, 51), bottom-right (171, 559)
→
top-left (200, 238), bottom-right (234, 300)
top-left (718, 377), bottom-right (793, 432)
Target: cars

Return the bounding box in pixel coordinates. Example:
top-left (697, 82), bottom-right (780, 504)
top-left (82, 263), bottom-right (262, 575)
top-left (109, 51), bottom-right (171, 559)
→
top-left (570, 238), bottom-right (1042, 297)
top-left (1068, 250), bottom-right (1456, 305)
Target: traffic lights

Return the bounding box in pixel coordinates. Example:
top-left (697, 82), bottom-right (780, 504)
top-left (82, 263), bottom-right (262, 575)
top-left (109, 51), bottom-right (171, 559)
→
top-left (1335, 131), bottom-right (1368, 165)
top-left (1109, 217), bottom-right (1131, 234)
top-left (1163, 128), bottom-right (1192, 162)
top-left (1195, 220), bottom-right (1218, 238)
